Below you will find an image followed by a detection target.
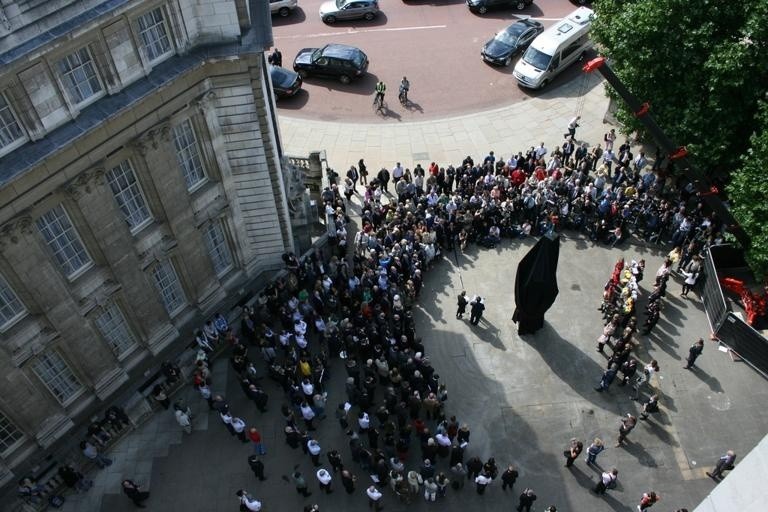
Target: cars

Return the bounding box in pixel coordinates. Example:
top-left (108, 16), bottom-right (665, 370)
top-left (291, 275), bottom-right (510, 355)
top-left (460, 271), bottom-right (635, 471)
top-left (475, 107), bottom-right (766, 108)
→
top-left (466, 0), bottom-right (610, 91)
top-left (268, 63), bottom-right (303, 102)
top-left (268, 0), bottom-right (298, 16)
top-left (317, 0), bottom-right (380, 24)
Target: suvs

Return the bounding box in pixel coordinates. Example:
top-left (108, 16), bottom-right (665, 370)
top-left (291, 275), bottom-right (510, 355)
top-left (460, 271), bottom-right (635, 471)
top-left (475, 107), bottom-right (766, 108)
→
top-left (294, 43), bottom-right (371, 86)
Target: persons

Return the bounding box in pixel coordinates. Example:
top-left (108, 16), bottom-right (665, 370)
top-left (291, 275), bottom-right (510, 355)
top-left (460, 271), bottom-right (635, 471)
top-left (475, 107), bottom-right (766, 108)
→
top-left (373, 82), bottom-right (385, 106)
top-left (273, 48), bottom-right (282, 68)
top-left (399, 77), bottom-right (409, 102)
